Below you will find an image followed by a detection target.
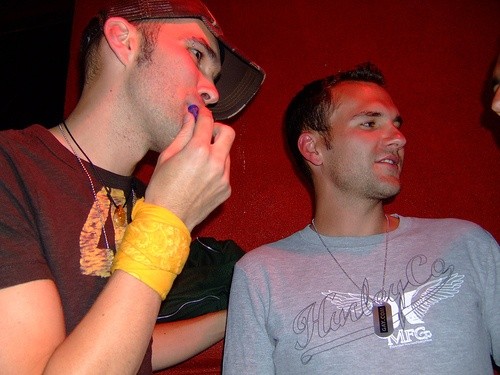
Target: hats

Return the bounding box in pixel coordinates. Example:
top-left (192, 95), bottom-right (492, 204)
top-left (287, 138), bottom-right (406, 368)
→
top-left (78, 0), bottom-right (266, 122)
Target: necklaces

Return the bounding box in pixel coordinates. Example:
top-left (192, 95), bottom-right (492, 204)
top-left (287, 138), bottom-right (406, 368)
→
top-left (57, 119), bottom-right (138, 267)
top-left (311, 215), bottom-right (393, 338)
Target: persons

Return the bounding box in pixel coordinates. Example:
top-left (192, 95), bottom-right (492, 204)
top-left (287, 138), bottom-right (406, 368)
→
top-left (1, 0), bottom-right (235, 375)
top-left (491, 60), bottom-right (499, 116)
top-left (222, 61), bottom-right (500, 375)
